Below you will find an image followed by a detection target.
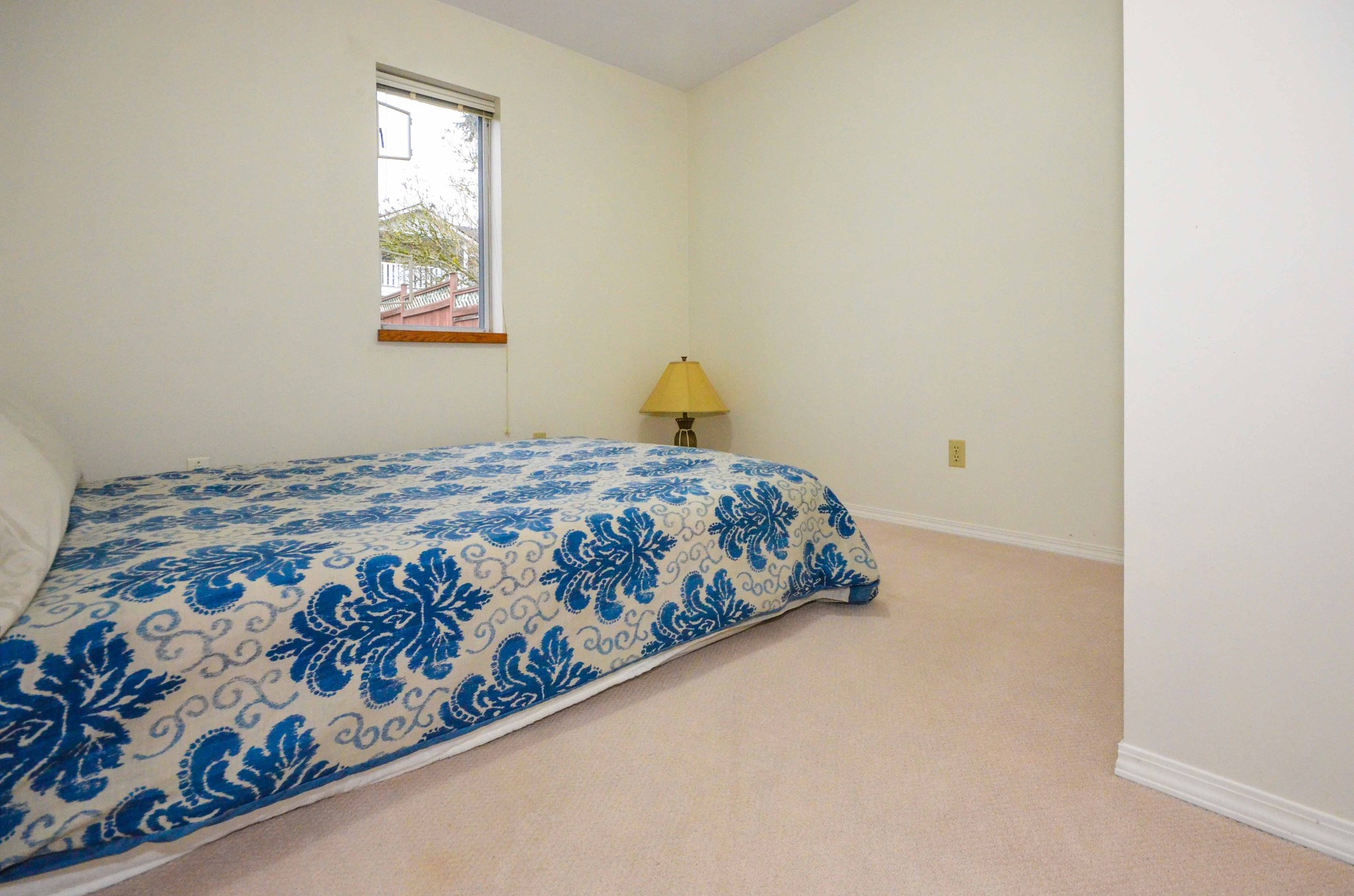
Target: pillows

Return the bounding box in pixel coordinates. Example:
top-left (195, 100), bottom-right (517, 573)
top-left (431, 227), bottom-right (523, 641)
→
top-left (0, 393), bottom-right (83, 640)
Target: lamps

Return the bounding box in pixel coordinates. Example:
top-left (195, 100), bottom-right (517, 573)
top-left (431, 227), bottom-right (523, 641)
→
top-left (640, 357), bottom-right (731, 449)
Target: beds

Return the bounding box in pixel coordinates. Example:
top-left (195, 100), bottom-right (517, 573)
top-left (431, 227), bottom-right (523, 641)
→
top-left (0, 436), bottom-right (880, 896)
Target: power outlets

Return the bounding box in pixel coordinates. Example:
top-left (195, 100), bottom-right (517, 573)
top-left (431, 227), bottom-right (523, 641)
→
top-left (948, 439), bottom-right (965, 466)
top-left (189, 458), bottom-right (209, 470)
